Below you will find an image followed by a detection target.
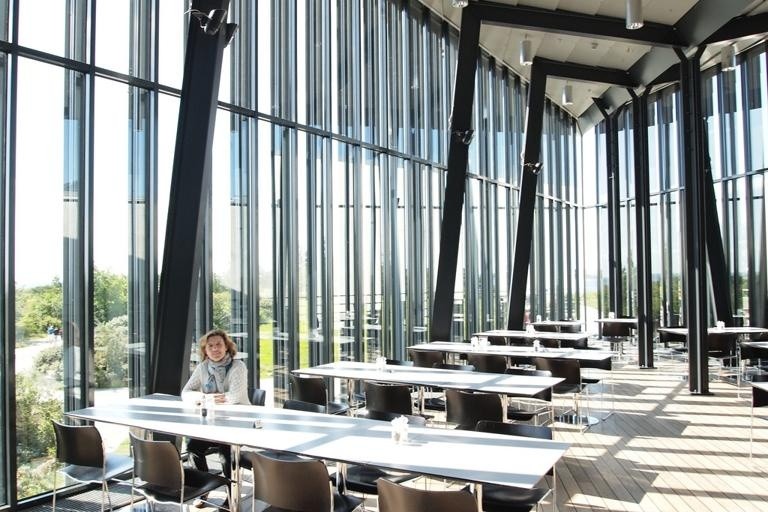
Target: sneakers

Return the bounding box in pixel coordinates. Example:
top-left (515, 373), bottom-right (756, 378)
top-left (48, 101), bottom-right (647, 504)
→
top-left (191, 492), bottom-right (210, 509)
top-left (218, 496), bottom-right (236, 512)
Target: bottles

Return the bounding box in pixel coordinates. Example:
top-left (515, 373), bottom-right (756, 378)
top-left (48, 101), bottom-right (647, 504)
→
top-left (533, 340), bottom-right (541, 353)
top-left (201, 393), bottom-right (209, 420)
top-left (391, 416), bottom-right (409, 443)
top-left (376, 354), bottom-right (388, 374)
top-left (470, 335), bottom-right (489, 349)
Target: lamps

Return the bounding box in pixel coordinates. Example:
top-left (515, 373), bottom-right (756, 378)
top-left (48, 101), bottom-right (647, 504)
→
top-left (191, 8), bottom-right (237, 48)
top-left (627, 0), bottom-right (645, 29)
top-left (456, 128), bottom-right (475, 145)
top-left (527, 161), bottom-right (543, 174)
top-left (563, 80), bottom-right (573, 105)
top-left (520, 31), bottom-right (534, 67)
top-left (720, 45), bottom-right (736, 71)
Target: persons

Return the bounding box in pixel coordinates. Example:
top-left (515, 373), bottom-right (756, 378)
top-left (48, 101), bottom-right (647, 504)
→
top-left (180, 329), bottom-right (252, 512)
top-left (47, 323), bottom-right (64, 344)
